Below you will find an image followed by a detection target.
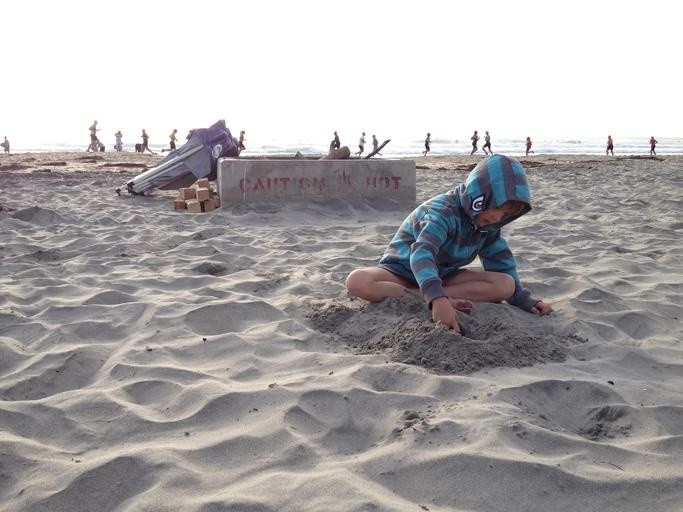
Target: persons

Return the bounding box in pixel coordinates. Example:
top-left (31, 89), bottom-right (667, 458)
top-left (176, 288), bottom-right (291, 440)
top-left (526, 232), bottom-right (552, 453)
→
top-left (86, 120), bottom-right (105, 152)
top-left (606, 136), bottom-right (614, 156)
top-left (141, 129), bottom-right (154, 154)
top-left (649, 137), bottom-right (658, 155)
top-left (3, 136), bottom-right (9, 154)
top-left (470, 131), bottom-right (493, 156)
top-left (114, 131), bottom-right (123, 152)
top-left (238, 131), bottom-right (246, 154)
top-left (355, 132), bottom-right (382, 157)
top-left (332, 132), bottom-right (340, 149)
top-left (345, 154), bottom-right (553, 333)
top-left (525, 137), bottom-right (534, 156)
top-left (186, 130), bottom-right (193, 140)
top-left (162, 129), bottom-right (178, 152)
top-left (422, 133), bottom-right (432, 156)
